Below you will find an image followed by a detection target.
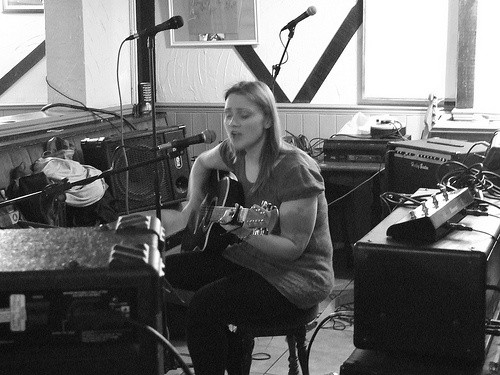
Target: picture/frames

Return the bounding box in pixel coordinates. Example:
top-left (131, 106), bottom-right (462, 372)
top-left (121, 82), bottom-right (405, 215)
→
top-left (168, 0), bottom-right (259, 47)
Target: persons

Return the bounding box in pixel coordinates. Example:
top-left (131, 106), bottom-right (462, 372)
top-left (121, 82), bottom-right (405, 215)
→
top-left (180, 82), bottom-right (334, 375)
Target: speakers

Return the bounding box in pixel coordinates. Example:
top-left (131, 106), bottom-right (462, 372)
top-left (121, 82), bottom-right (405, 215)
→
top-left (386, 138), bottom-right (490, 207)
top-left (0, 223), bottom-right (164, 374)
top-left (81, 126), bottom-right (192, 218)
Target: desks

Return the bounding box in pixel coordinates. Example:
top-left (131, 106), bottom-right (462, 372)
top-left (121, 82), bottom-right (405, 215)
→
top-left (316, 161), bottom-right (386, 267)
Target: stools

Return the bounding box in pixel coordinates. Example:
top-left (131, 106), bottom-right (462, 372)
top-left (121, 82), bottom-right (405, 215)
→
top-left (231, 304), bottom-right (320, 375)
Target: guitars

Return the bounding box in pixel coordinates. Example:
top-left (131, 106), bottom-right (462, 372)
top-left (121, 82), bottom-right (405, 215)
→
top-left (179, 176), bottom-right (280, 255)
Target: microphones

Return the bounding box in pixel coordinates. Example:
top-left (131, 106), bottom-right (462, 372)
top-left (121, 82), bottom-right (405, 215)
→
top-left (126, 16), bottom-right (184, 41)
top-left (158, 130), bottom-right (216, 151)
top-left (281, 7), bottom-right (316, 30)
